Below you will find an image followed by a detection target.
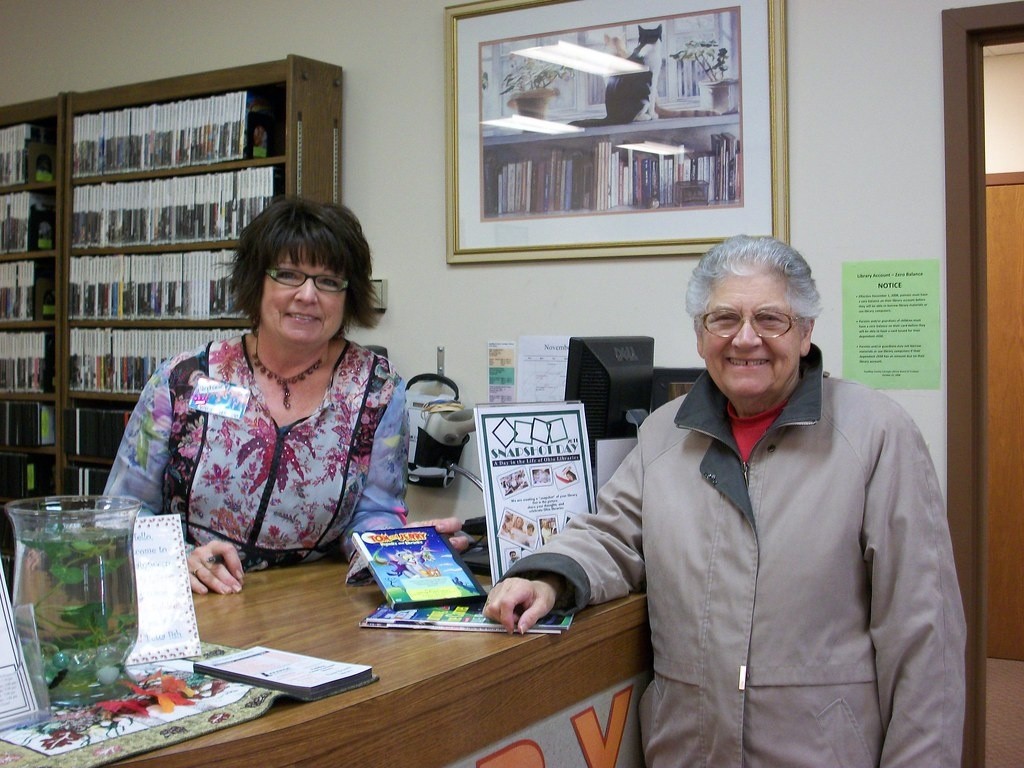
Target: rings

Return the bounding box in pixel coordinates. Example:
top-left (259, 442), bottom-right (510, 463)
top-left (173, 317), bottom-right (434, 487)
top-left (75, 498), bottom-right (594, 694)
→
top-left (486, 600), bottom-right (490, 605)
top-left (193, 564), bottom-right (204, 576)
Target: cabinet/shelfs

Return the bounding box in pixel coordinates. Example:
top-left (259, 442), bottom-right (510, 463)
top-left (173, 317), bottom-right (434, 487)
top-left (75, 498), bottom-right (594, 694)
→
top-left (62, 54), bottom-right (345, 486)
top-left (0, 88), bottom-right (64, 556)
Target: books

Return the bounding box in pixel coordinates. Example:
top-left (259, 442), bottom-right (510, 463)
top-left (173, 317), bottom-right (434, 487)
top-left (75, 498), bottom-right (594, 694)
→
top-left (350, 527), bottom-right (489, 610)
top-left (192, 646), bottom-right (372, 699)
top-left (0, 91), bottom-right (286, 514)
top-left (497, 131), bottom-right (742, 214)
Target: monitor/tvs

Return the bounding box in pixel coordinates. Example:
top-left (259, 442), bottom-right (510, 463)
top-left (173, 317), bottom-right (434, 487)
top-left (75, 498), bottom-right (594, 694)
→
top-left (565, 336), bottom-right (654, 467)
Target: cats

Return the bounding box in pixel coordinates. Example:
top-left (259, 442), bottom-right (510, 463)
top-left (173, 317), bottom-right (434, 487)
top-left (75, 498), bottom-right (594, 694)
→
top-left (600, 33), bottom-right (722, 117)
top-left (566, 23), bottom-right (664, 127)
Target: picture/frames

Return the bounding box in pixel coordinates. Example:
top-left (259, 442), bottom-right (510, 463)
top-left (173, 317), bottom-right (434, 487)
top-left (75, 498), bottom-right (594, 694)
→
top-left (441, 0), bottom-right (792, 273)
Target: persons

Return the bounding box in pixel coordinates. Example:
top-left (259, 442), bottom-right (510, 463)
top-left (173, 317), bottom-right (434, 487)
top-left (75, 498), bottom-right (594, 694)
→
top-left (508, 551), bottom-right (517, 569)
top-left (555, 470), bottom-right (576, 484)
top-left (501, 470), bottom-right (527, 495)
top-left (481, 236), bottom-right (967, 768)
top-left (97, 195), bottom-right (469, 594)
top-left (503, 512), bottom-right (534, 547)
top-left (541, 520), bottom-right (552, 545)
top-left (533, 469), bottom-right (550, 484)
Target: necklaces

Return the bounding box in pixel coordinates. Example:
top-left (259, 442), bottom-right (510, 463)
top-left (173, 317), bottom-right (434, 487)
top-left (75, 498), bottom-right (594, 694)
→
top-left (253, 333), bottom-right (322, 409)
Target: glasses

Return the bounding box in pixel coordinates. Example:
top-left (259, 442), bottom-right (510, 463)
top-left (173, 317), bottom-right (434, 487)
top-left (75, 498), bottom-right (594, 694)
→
top-left (265, 267), bottom-right (348, 293)
top-left (699, 309), bottom-right (805, 338)
